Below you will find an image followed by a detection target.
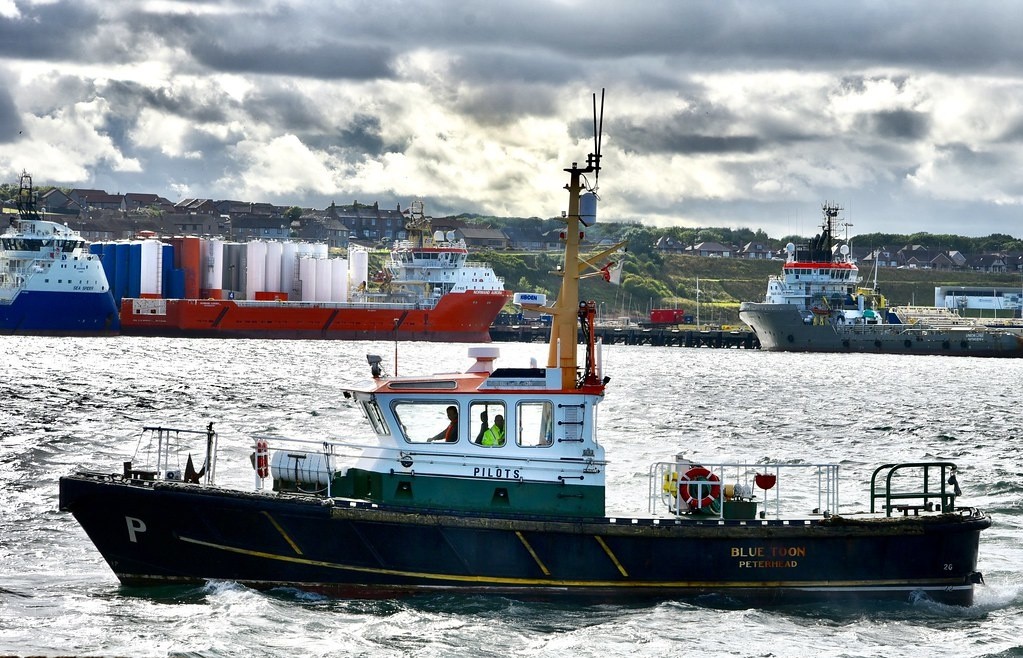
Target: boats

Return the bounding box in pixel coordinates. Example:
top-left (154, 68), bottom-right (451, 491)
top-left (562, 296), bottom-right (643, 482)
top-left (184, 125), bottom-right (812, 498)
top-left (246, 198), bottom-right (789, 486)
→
top-left (738, 199), bottom-right (1023, 358)
top-left (0, 165), bottom-right (514, 343)
top-left (58, 86), bottom-right (994, 608)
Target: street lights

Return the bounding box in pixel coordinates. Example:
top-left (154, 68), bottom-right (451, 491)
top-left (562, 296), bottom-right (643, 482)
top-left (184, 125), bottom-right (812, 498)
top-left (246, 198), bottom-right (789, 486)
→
top-left (845, 222), bottom-right (854, 245)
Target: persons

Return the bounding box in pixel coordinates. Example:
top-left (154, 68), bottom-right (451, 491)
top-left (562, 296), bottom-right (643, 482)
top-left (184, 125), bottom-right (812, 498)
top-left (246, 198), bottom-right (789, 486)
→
top-left (426, 406), bottom-right (506, 447)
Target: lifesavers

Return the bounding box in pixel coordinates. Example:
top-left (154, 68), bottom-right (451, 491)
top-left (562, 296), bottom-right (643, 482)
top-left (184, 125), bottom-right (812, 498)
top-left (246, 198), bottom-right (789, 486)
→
top-left (257, 443), bottom-right (269, 479)
top-left (904, 340), bottom-right (910, 346)
top-left (943, 341), bottom-right (949, 349)
top-left (680, 467), bottom-right (720, 508)
top-left (788, 335), bottom-right (793, 342)
top-left (875, 341), bottom-right (882, 347)
